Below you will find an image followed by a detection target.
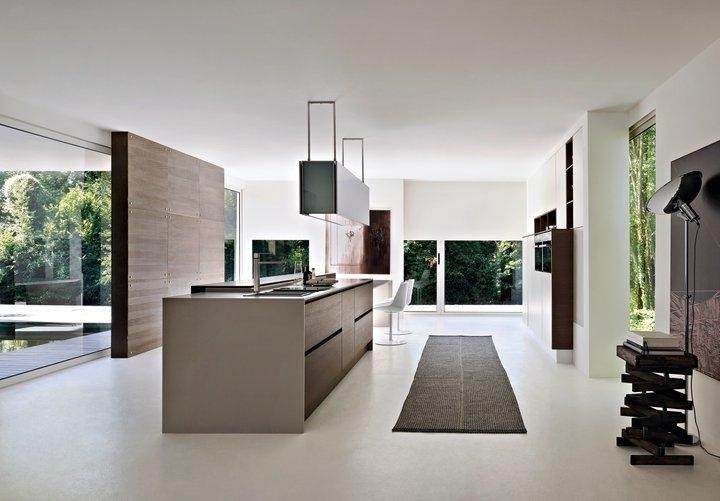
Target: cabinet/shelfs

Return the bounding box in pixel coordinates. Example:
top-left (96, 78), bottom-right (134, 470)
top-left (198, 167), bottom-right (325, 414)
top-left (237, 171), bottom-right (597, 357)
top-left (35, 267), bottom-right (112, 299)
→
top-left (520, 225), bottom-right (575, 350)
top-left (163, 281), bottom-right (373, 434)
top-left (611, 345), bottom-right (702, 467)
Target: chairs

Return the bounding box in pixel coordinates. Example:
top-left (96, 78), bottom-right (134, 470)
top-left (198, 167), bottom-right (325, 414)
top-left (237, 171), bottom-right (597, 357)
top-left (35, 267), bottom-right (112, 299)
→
top-left (373, 278), bottom-right (416, 346)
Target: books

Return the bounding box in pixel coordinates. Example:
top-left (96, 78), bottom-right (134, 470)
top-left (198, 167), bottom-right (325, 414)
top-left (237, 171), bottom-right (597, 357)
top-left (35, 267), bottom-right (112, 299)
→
top-left (624, 328), bottom-right (685, 356)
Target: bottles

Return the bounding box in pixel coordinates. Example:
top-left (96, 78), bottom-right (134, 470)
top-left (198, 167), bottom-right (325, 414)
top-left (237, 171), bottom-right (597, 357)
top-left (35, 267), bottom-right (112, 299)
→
top-left (303, 265), bottom-right (312, 285)
top-left (312, 268), bottom-right (316, 279)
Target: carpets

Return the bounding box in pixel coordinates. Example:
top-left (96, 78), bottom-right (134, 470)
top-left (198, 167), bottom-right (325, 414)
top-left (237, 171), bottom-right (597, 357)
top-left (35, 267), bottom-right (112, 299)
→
top-left (393, 335), bottom-right (530, 436)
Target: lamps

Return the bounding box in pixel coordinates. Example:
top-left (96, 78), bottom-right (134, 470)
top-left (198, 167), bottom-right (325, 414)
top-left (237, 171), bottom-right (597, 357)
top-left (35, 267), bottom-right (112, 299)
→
top-left (645, 168), bottom-right (720, 461)
top-left (298, 100), bottom-right (372, 231)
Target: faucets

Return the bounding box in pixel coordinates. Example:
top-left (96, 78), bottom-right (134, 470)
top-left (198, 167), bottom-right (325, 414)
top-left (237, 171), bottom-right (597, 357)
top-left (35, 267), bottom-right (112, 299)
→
top-left (252, 252), bottom-right (274, 292)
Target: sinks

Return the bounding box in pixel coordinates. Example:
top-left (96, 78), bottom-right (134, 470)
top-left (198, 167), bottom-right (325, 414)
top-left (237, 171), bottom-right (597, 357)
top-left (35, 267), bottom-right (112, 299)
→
top-left (242, 290), bottom-right (313, 297)
